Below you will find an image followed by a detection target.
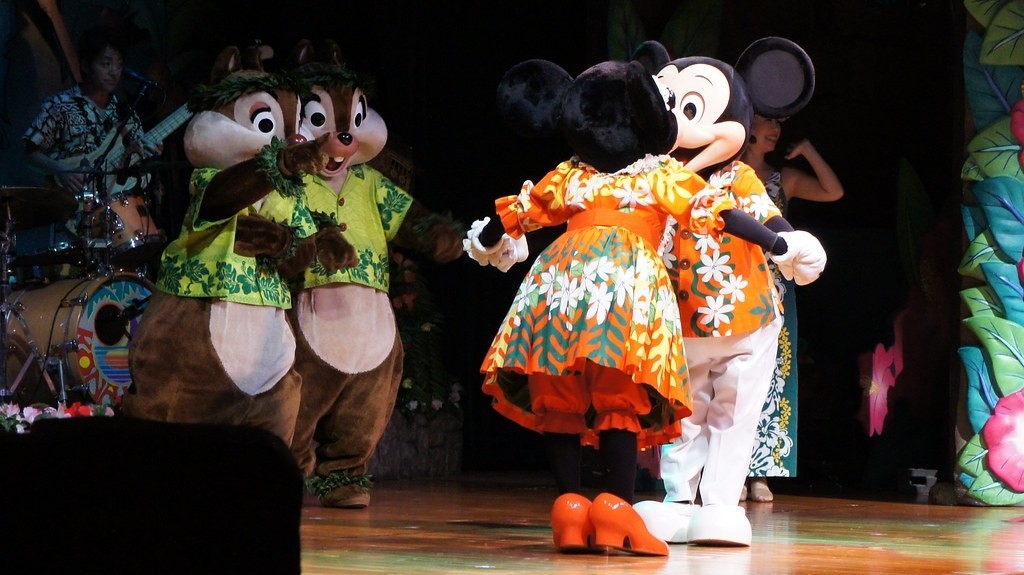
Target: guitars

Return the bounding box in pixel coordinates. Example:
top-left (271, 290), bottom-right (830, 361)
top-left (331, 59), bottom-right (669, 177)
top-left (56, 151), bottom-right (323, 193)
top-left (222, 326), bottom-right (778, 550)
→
top-left (53, 100), bottom-right (195, 211)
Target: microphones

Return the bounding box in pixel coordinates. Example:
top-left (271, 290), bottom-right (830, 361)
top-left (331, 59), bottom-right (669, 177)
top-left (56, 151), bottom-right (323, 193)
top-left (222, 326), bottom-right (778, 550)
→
top-left (122, 67), bottom-right (159, 86)
top-left (116, 154), bottom-right (132, 185)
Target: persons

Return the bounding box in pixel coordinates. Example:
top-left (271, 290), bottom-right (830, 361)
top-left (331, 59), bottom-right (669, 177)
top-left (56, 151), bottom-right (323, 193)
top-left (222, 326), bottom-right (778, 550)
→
top-left (20, 37), bottom-right (162, 262)
top-left (739, 110), bottom-right (841, 501)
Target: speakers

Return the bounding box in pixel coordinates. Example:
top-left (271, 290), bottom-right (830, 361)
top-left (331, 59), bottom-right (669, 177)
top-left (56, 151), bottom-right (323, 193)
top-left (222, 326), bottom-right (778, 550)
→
top-left (0, 416), bottom-right (305, 575)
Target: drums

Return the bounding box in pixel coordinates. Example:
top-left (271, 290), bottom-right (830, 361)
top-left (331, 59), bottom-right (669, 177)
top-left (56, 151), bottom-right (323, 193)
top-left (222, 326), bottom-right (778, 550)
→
top-left (13, 240), bottom-right (94, 289)
top-left (1, 268), bottom-right (161, 409)
top-left (82, 189), bottom-right (165, 269)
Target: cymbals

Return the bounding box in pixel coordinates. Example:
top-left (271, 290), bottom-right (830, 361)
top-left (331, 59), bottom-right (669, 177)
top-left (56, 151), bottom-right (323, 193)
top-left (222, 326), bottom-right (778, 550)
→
top-left (1, 184), bottom-right (80, 234)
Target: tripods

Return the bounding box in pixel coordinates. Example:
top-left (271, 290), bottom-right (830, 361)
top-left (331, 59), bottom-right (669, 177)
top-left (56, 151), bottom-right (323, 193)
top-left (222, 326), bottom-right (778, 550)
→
top-left (0, 192), bottom-right (60, 411)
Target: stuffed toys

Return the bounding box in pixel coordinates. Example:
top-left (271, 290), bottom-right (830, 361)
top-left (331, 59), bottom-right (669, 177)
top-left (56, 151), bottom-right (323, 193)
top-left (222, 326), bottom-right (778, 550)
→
top-left (136, 38), bottom-right (468, 508)
top-left (462, 37), bottom-right (827, 559)
